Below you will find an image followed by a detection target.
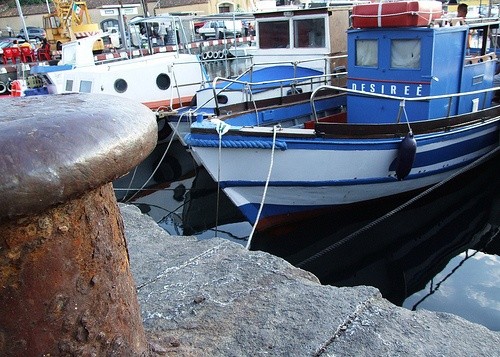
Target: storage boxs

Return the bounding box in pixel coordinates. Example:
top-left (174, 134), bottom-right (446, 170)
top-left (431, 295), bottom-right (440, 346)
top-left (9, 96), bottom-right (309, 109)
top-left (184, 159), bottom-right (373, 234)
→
top-left (352, 1), bottom-right (442, 27)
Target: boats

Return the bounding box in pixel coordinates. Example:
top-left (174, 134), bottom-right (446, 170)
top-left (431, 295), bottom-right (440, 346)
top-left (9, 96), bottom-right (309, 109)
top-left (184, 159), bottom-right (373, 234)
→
top-left (0, 0), bottom-right (256, 145)
top-left (163, 0), bottom-right (500, 232)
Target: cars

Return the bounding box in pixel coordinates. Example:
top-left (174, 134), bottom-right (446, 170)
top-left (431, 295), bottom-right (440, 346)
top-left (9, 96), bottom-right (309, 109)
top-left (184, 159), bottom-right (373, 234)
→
top-left (15, 26), bottom-right (46, 41)
top-left (198, 20), bottom-right (242, 39)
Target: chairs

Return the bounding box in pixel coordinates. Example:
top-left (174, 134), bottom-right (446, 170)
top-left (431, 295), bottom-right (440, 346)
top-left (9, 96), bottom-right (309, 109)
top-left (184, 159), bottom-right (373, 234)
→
top-left (2, 44), bottom-right (51, 64)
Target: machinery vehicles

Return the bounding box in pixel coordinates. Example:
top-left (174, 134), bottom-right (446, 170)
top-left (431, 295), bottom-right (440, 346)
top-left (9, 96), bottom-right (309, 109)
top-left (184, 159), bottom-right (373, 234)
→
top-left (40, 0), bottom-right (105, 58)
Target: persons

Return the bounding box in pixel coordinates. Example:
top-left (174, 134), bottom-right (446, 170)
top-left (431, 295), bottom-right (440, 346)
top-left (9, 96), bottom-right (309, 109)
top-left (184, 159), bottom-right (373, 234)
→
top-left (449, 2), bottom-right (472, 56)
top-left (242, 17), bottom-right (253, 36)
top-left (219, 30), bottom-right (224, 38)
top-left (0, 23), bottom-right (50, 61)
top-left (139, 23), bottom-right (180, 45)
top-left (108, 29), bottom-right (119, 45)
